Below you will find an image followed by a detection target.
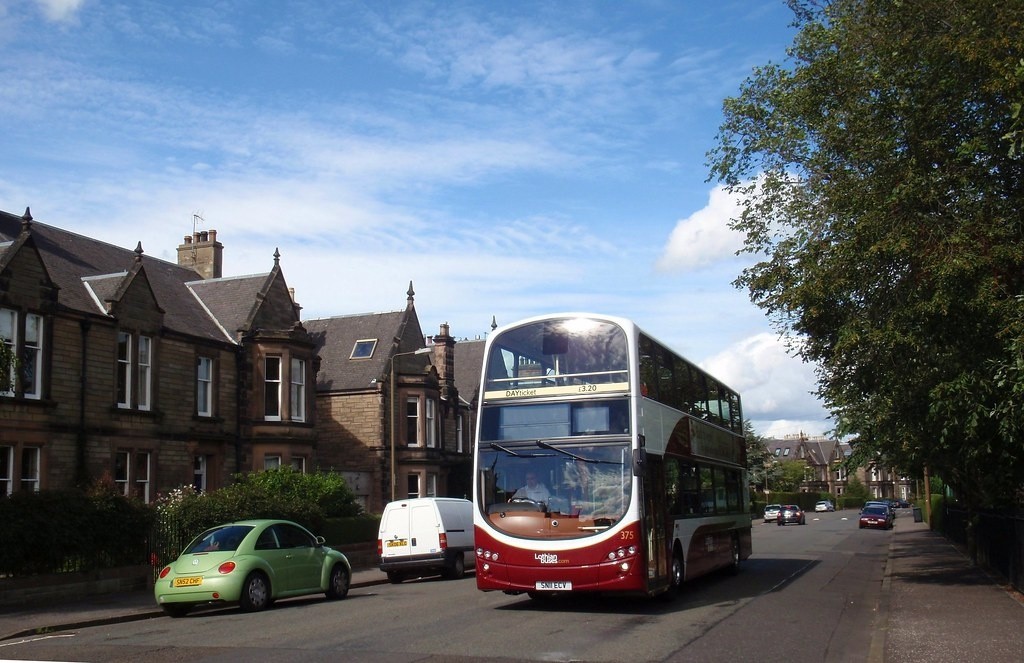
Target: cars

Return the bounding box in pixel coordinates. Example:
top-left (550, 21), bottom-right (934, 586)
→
top-left (763, 504), bottom-right (781, 524)
top-left (777, 505), bottom-right (805, 526)
top-left (153, 518), bottom-right (351, 617)
top-left (861, 493), bottom-right (910, 519)
top-left (815, 501), bottom-right (835, 513)
top-left (858, 506), bottom-right (891, 529)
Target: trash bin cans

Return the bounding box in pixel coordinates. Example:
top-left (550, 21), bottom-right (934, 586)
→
top-left (913, 507), bottom-right (924, 522)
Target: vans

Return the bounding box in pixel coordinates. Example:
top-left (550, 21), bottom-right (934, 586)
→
top-left (378, 498), bottom-right (472, 583)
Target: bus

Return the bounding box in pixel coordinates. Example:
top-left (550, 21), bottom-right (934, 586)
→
top-left (470, 312), bottom-right (755, 605)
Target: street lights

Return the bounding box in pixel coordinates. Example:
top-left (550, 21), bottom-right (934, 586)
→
top-left (390, 347), bottom-right (432, 500)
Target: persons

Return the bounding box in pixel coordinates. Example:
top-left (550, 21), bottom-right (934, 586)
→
top-left (593, 499), bottom-right (621, 522)
top-left (507, 470), bottom-right (551, 507)
